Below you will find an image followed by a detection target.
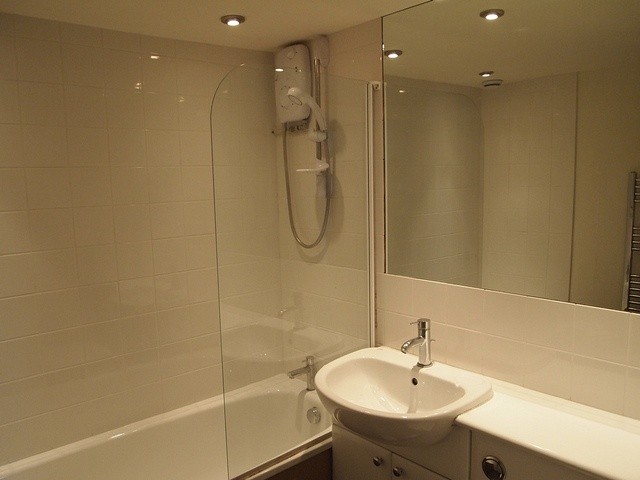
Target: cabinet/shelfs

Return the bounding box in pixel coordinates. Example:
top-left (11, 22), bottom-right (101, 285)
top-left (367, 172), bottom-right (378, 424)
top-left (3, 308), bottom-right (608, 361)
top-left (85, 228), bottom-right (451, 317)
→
top-left (333, 417), bottom-right (471, 480)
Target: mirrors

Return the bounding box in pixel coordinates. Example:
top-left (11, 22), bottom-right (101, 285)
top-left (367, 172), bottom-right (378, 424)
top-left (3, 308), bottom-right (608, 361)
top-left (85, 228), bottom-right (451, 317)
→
top-left (381, 0), bottom-right (639, 312)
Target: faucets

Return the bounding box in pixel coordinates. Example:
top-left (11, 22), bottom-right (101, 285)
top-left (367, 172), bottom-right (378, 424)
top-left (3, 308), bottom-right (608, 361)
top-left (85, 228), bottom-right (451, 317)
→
top-left (288, 356), bottom-right (316, 392)
top-left (401, 318), bottom-right (436, 368)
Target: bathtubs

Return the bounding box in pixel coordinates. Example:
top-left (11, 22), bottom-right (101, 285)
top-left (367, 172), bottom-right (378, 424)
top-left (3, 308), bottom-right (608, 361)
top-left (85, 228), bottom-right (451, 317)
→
top-left (1, 370), bottom-right (333, 480)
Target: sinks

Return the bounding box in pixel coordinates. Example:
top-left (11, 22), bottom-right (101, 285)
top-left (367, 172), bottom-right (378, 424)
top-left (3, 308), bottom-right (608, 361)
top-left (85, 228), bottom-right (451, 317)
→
top-left (327, 360), bottom-right (464, 414)
